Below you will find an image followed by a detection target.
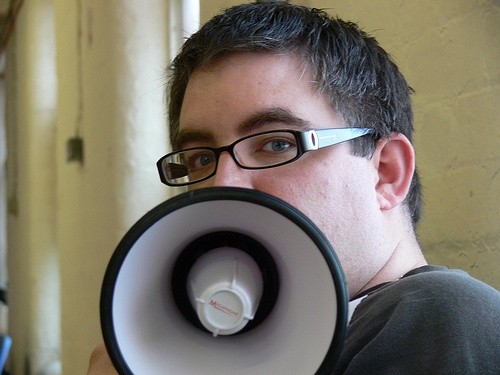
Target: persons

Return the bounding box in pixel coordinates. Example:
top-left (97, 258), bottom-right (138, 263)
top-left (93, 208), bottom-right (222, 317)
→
top-left (87, 0), bottom-right (499, 374)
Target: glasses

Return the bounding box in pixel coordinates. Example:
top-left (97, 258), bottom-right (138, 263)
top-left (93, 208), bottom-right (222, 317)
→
top-left (156, 127), bottom-right (372, 187)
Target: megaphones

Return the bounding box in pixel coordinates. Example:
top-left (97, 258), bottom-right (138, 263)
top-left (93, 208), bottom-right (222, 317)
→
top-left (99, 188), bottom-right (348, 375)
